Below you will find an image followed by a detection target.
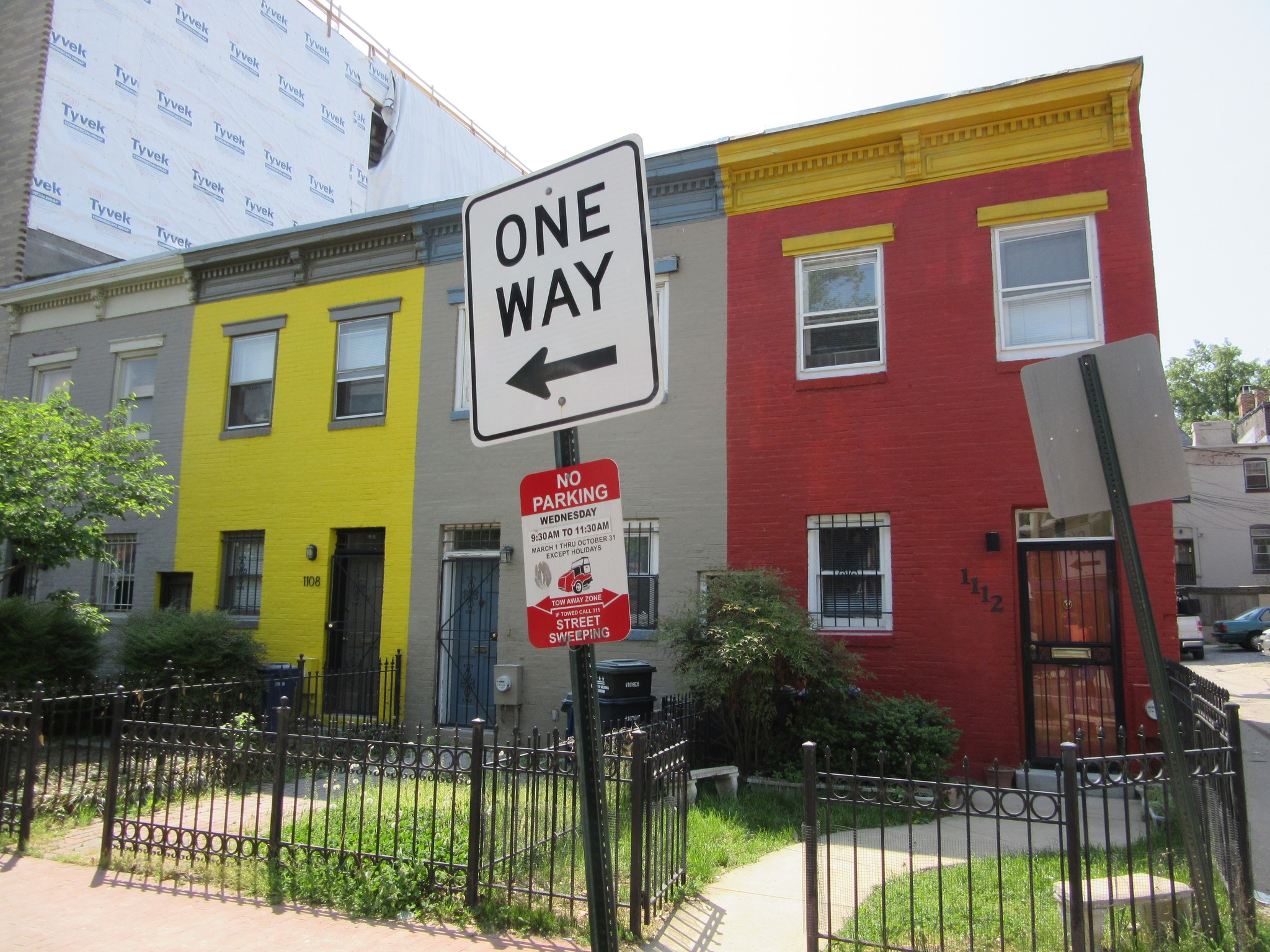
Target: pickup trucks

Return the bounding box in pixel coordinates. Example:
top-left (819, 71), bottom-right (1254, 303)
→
top-left (1176, 591), bottom-right (1205, 660)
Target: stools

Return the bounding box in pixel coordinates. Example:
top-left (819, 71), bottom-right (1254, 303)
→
top-left (1051, 870), bottom-right (1196, 952)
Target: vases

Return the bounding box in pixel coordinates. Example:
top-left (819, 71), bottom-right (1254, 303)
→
top-left (982, 764), bottom-right (1017, 789)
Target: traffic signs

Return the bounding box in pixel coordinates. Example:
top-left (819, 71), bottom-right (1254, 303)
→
top-left (460, 133), bottom-right (666, 450)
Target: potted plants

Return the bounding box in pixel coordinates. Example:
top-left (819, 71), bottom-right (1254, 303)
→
top-left (1134, 782), bottom-right (1179, 830)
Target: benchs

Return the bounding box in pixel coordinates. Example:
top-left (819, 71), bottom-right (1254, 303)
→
top-left (661, 765), bottom-right (740, 806)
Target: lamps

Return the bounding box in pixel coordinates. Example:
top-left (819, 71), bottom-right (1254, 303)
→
top-left (498, 544), bottom-right (513, 564)
top-left (306, 544), bottom-right (317, 560)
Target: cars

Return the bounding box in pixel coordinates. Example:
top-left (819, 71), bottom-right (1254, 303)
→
top-left (1210, 605), bottom-right (1270, 656)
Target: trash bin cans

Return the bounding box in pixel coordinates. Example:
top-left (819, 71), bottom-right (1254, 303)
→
top-left (560, 658), bottom-right (658, 763)
top-left (253, 663), bottom-right (298, 732)
